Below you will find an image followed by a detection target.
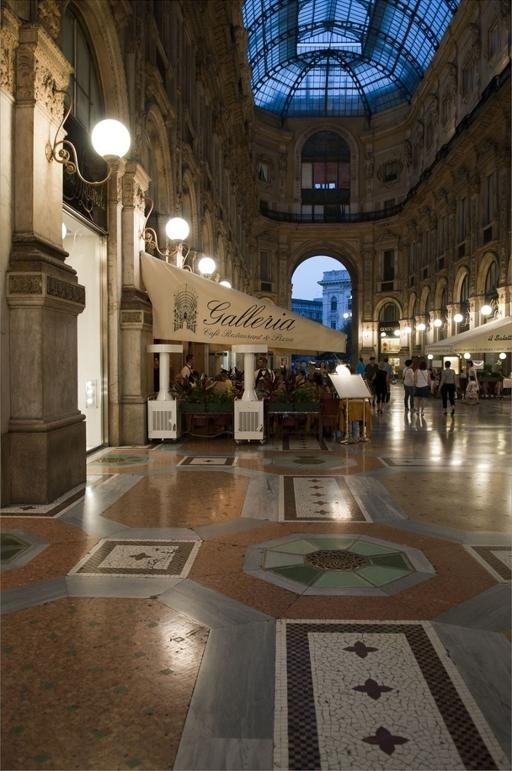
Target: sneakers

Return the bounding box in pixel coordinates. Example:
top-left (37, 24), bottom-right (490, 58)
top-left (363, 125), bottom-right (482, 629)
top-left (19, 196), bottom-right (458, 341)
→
top-left (405, 407), bottom-right (417, 411)
top-left (442, 406), bottom-right (456, 414)
top-left (377, 408), bottom-right (384, 415)
top-left (415, 412), bottom-right (425, 415)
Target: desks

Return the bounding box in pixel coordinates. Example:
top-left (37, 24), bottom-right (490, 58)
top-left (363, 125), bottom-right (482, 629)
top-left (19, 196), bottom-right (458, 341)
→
top-left (267, 409), bottom-right (319, 435)
top-left (184, 410), bottom-right (235, 439)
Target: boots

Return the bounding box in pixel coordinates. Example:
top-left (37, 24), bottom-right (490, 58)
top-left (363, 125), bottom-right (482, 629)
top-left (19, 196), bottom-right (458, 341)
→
top-left (369, 400), bottom-right (376, 407)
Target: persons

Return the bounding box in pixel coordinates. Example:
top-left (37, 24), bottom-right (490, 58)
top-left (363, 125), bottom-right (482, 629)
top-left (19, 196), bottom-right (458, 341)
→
top-left (461, 360), bottom-right (480, 404)
top-left (181, 352), bottom-right (331, 392)
top-left (438, 361), bottom-right (457, 415)
top-left (356, 357), bottom-right (432, 416)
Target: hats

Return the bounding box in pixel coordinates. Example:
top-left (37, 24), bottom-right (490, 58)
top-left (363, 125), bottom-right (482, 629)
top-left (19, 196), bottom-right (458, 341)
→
top-left (369, 357), bottom-right (376, 360)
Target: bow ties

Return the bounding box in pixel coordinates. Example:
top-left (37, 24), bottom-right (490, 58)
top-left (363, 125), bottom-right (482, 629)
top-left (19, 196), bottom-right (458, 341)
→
top-left (260, 369), bottom-right (267, 371)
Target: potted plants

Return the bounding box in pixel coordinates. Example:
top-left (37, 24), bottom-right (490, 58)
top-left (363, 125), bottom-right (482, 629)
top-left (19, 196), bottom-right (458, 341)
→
top-left (266, 383), bottom-right (289, 411)
top-left (290, 382), bottom-right (320, 411)
top-left (168, 373), bottom-right (233, 427)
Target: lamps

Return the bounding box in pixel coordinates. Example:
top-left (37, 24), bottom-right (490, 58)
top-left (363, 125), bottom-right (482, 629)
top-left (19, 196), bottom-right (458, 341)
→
top-left (140, 216), bottom-right (189, 257)
top-left (219, 281), bottom-right (230, 288)
top-left (393, 304), bottom-right (502, 337)
top-left (45, 118), bottom-right (132, 188)
top-left (182, 257), bottom-right (215, 278)
